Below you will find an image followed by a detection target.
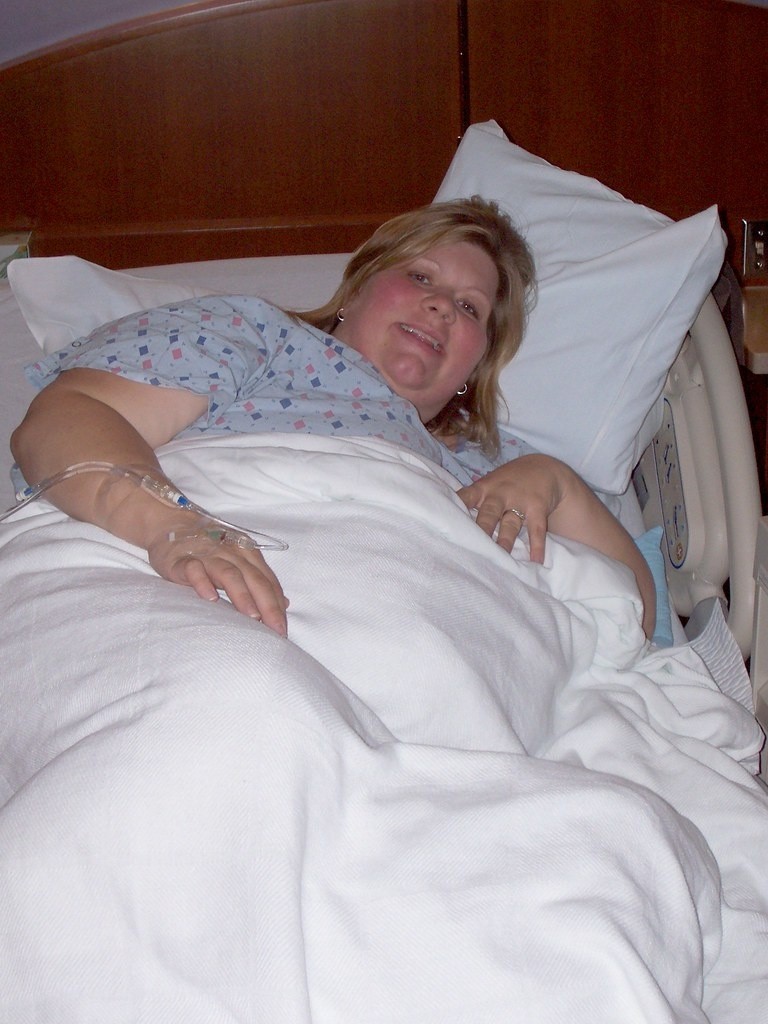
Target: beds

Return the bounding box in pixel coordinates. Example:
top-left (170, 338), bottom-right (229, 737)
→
top-left (0, 0), bottom-right (768, 1023)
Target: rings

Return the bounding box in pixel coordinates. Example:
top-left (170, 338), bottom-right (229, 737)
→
top-left (502, 508), bottom-right (524, 521)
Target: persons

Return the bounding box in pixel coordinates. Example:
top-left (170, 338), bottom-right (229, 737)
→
top-left (8, 194), bottom-right (657, 642)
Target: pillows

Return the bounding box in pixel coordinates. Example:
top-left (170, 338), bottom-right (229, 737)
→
top-left (430, 118), bottom-right (727, 498)
